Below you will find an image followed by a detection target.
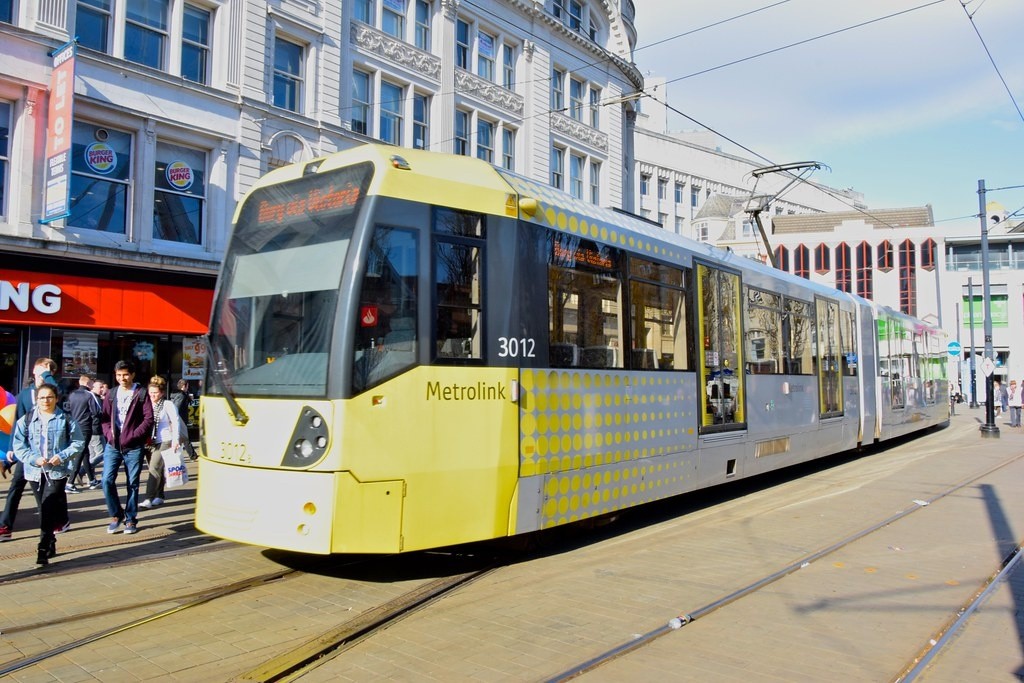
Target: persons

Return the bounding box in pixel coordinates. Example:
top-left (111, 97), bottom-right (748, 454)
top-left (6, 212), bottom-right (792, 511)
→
top-left (948, 380), bottom-right (963, 406)
top-left (994, 380), bottom-right (1024, 427)
top-left (0, 357), bottom-right (199, 569)
top-left (353, 276), bottom-right (418, 386)
top-left (442, 322), bottom-right (472, 357)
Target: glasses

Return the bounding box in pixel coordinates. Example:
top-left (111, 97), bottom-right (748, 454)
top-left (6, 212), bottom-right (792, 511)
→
top-left (36, 396), bottom-right (57, 402)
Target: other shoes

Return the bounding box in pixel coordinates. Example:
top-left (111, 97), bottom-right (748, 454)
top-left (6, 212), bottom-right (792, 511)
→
top-left (1011, 425), bottom-right (1016, 427)
top-left (151, 497), bottom-right (163, 506)
top-left (8, 466), bottom-right (11, 474)
top-left (77, 474), bottom-right (83, 486)
top-left (36, 550), bottom-right (48, 564)
top-left (0, 460), bottom-right (6, 479)
top-left (138, 499), bottom-right (152, 509)
top-left (1016, 423), bottom-right (1021, 427)
top-left (47, 543), bottom-right (55, 558)
top-left (191, 455), bottom-right (199, 462)
top-left (996, 416), bottom-right (1002, 418)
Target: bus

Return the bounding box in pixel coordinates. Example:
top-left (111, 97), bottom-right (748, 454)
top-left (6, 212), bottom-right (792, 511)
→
top-left (192, 144), bottom-right (951, 556)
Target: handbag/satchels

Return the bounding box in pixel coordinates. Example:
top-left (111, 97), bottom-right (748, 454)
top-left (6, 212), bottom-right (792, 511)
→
top-left (160, 447), bottom-right (189, 488)
top-left (166, 402), bottom-right (188, 443)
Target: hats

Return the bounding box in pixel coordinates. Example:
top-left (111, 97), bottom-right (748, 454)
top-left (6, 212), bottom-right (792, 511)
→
top-left (1009, 380), bottom-right (1016, 384)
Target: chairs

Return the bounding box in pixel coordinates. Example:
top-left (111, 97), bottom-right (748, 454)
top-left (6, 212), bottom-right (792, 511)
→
top-left (549, 342), bottom-right (673, 369)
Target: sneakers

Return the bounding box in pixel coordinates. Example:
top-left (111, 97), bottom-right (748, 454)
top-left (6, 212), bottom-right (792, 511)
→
top-left (107, 509), bottom-right (125, 533)
top-left (64, 485), bottom-right (81, 494)
top-left (124, 521), bottom-right (136, 534)
top-left (52, 521), bottom-right (70, 533)
top-left (89, 480), bottom-right (102, 489)
top-left (0, 526), bottom-right (11, 539)
